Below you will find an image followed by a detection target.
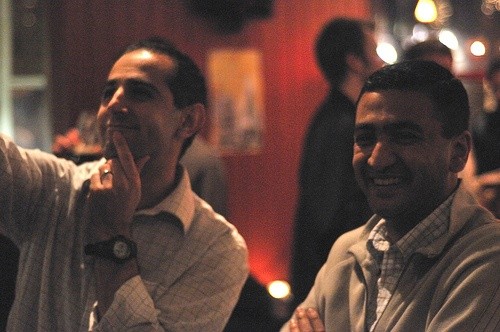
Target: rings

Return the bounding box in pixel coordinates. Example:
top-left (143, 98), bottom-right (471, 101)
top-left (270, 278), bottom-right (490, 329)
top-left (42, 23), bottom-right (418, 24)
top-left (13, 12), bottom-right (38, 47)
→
top-left (101, 170), bottom-right (112, 178)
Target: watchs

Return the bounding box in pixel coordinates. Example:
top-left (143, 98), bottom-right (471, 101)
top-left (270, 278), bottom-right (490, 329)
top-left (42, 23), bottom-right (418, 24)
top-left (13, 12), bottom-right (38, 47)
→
top-left (84, 235), bottom-right (138, 264)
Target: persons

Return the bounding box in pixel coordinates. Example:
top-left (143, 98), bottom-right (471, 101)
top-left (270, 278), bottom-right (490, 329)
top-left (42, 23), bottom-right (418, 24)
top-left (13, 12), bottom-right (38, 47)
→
top-left (283, 58), bottom-right (500, 332)
top-left (462, 56), bottom-right (500, 220)
top-left (0, 37), bottom-right (250, 332)
top-left (406, 39), bottom-right (453, 73)
top-left (289, 15), bottom-right (386, 313)
